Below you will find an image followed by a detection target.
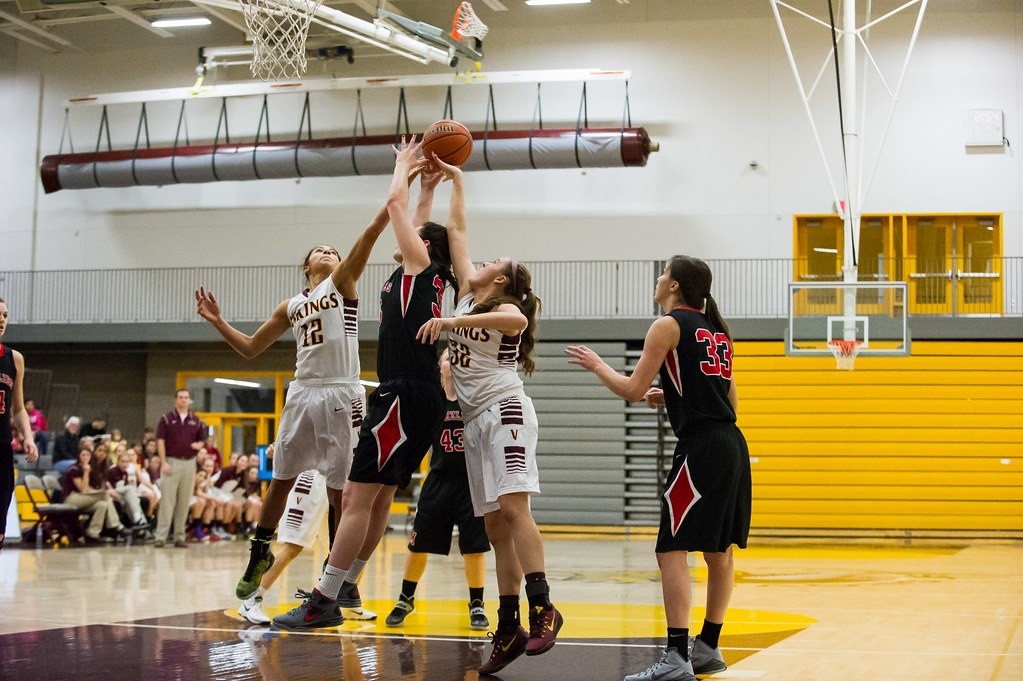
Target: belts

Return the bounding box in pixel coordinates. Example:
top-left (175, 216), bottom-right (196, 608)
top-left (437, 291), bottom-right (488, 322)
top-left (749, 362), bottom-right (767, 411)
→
top-left (167, 455), bottom-right (194, 460)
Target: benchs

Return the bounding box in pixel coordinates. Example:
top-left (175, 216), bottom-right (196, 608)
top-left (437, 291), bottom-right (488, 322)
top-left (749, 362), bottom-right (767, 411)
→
top-left (13, 441), bottom-right (53, 486)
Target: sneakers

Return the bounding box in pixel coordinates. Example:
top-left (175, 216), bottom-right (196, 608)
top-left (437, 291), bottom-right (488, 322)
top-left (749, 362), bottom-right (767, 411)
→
top-left (339, 607), bottom-right (377, 620)
top-left (386, 593), bottom-right (415, 626)
top-left (238, 592), bottom-right (271, 624)
top-left (272, 587), bottom-right (344, 631)
top-left (478, 626), bottom-right (528, 675)
top-left (624, 646), bottom-right (697, 681)
top-left (323, 557), bottom-right (361, 607)
top-left (525, 603), bottom-right (563, 655)
top-left (688, 634), bottom-right (727, 675)
top-left (468, 599), bottom-right (490, 630)
top-left (236, 536), bottom-right (275, 600)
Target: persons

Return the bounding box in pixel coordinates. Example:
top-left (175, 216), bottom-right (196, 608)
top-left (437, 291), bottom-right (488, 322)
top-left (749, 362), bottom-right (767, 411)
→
top-left (564, 256), bottom-right (753, 681)
top-left (80, 418), bottom-right (263, 544)
top-left (416, 152), bottom-right (564, 674)
top-left (0, 298), bottom-right (39, 550)
top-left (270, 132), bottom-right (451, 633)
top-left (154, 389), bottom-right (208, 547)
top-left (387, 347), bottom-right (490, 629)
top-left (195, 156), bottom-right (429, 598)
top-left (11, 397), bottom-right (51, 455)
top-left (63, 446), bottom-right (132, 545)
top-left (52, 417), bottom-right (80, 473)
top-left (236, 443), bottom-right (377, 623)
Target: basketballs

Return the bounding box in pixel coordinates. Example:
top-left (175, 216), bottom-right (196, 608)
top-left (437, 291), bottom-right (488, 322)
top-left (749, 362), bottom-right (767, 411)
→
top-left (421, 119), bottom-right (473, 170)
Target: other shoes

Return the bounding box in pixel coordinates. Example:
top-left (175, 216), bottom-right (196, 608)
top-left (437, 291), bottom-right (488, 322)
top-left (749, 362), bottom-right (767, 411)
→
top-left (117, 526), bottom-right (133, 535)
top-left (175, 541), bottom-right (188, 547)
top-left (186, 525), bottom-right (250, 542)
top-left (154, 541), bottom-right (164, 547)
top-left (89, 538), bottom-right (107, 548)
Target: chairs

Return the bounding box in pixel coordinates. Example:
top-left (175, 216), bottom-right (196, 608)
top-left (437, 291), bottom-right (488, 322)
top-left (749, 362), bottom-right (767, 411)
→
top-left (23, 474), bottom-right (94, 545)
